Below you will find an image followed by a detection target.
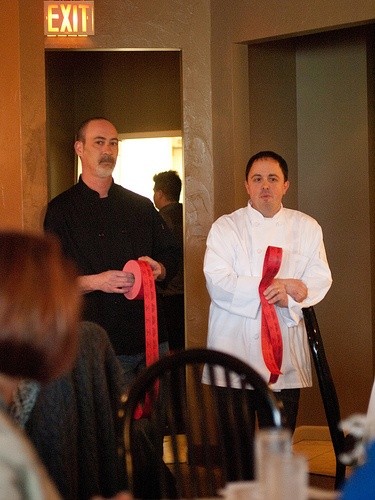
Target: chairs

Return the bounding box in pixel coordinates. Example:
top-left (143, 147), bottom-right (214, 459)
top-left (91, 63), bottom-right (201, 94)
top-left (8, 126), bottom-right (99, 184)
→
top-left (26, 305), bottom-right (375, 500)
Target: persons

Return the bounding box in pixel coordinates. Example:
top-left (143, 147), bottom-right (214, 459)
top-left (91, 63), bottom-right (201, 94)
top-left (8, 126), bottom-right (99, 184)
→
top-left (31, 317), bottom-right (125, 500)
top-left (0, 230), bottom-right (83, 499)
top-left (153, 170), bottom-right (184, 353)
top-left (201, 150), bottom-right (333, 459)
top-left (42, 118), bottom-right (182, 456)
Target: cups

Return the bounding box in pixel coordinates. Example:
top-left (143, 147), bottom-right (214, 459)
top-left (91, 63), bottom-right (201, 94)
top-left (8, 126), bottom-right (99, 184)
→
top-left (255, 431), bottom-right (293, 500)
top-left (215, 481), bottom-right (254, 499)
top-left (292, 457), bottom-right (308, 500)
